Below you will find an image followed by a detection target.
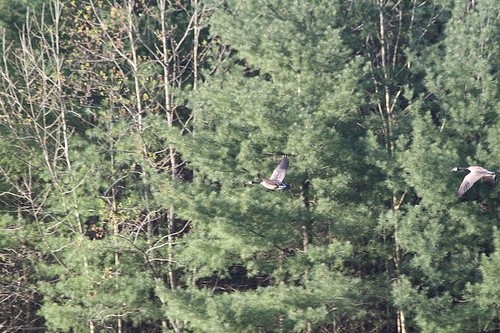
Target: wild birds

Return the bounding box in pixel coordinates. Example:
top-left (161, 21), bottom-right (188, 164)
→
top-left (245, 155), bottom-right (290, 191)
top-left (450, 166), bottom-right (500, 199)
